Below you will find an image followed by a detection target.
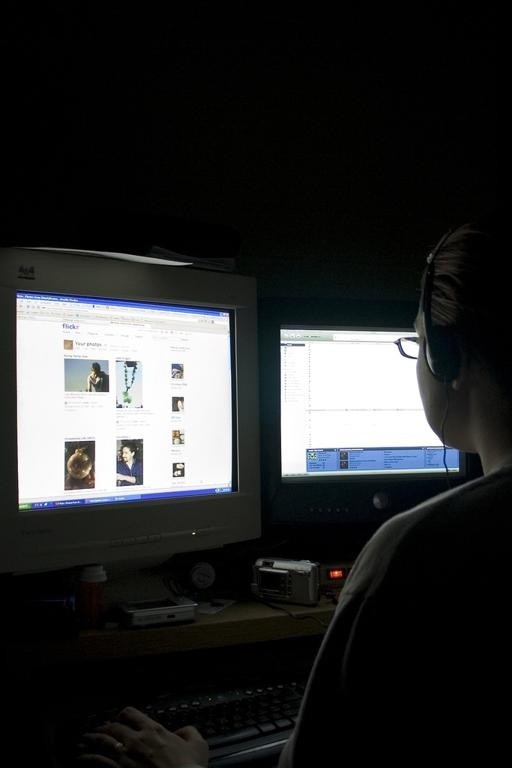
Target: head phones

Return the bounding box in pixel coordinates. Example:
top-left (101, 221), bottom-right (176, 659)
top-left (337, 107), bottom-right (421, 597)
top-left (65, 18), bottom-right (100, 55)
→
top-left (419, 226), bottom-right (465, 384)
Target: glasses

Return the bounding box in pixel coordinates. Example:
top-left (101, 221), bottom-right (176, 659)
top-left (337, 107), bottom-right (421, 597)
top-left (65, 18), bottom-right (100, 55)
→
top-left (394, 336), bottom-right (423, 359)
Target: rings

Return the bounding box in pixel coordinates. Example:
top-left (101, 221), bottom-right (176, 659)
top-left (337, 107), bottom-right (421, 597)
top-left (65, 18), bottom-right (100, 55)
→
top-left (112, 742), bottom-right (124, 759)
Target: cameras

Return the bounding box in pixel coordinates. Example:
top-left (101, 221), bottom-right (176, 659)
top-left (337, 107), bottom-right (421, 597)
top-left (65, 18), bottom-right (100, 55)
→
top-left (252, 557), bottom-right (324, 607)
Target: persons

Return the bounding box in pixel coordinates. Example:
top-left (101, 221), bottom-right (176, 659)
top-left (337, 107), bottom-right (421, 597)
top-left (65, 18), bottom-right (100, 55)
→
top-left (79, 214), bottom-right (511, 768)
top-left (116, 442), bottom-right (143, 486)
top-left (88, 362), bottom-right (109, 392)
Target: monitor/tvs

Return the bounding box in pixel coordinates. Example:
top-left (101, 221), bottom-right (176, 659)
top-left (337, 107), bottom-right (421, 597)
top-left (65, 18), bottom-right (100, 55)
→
top-left (275, 326), bottom-right (469, 518)
top-left (0, 248), bottom-right (261, 606)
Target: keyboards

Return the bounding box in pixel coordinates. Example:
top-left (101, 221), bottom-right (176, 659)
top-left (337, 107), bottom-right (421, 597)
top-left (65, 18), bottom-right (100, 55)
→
top-left (140, 681), bottom-right (305, 761)
